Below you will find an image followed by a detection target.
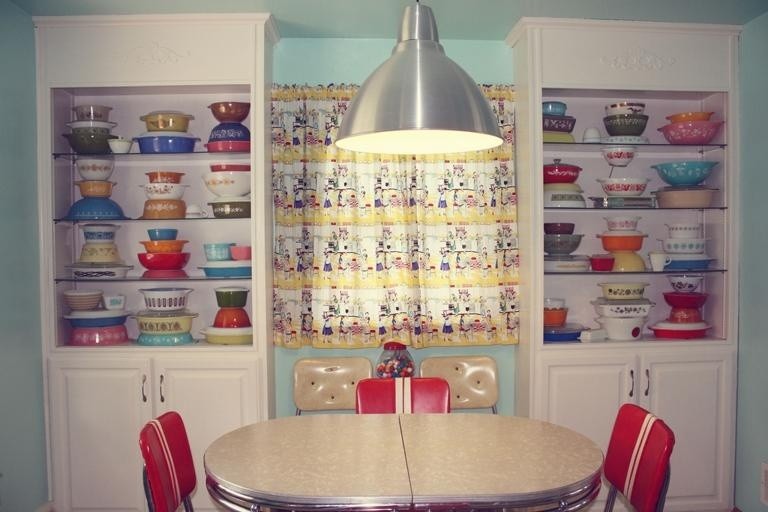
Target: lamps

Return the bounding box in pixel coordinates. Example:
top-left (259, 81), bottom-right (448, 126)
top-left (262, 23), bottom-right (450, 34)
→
top-left (328, 0), bottom-right (505, 157)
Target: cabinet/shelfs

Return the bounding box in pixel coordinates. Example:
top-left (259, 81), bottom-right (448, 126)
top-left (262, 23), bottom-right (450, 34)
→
top-left (28, 10), bottom-right (282, 512)
top-left (506, 13), bottom-right (745, 512)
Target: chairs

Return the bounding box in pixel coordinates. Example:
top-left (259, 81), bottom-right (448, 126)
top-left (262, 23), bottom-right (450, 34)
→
top-left (597, 399), bottom-right (676, 512)
top-left (421, 353), bottom-right (504, 417)
top-left (350, 374), bottom-right (454, 418)
top-left (292, 353), bottom-right (373, 418)
top-left (138, 409), bottom-right (206, 511)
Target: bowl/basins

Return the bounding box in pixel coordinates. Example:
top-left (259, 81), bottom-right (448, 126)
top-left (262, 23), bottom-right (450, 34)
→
top-left (542, 144), bottom-right (724, 208)
top-left (61, 287), bottom-right (252, 345)
top-left (578, 275), bottom-right (714, 342)
top-left (542, 213), bottom-right (721, 269)
top-left (543, 97), bottom-right (723, 144)
top-left (542, 298), bottom-right (595, 342)
top-left (59, 221), bottom-right (190, 278)
top-left (61, 100), bottom-right (252, 152)
top-left (60, 155), bottom-right (249, 216)
top-left (196, 243), bottom-right (251, 277)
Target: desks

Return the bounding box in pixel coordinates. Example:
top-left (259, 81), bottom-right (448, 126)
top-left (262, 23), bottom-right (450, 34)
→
top-left (198, 408), bottom-right (604, 512)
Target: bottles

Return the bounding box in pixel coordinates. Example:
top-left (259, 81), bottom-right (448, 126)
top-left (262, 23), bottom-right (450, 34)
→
top-left (376, 341), bottom-right (416, 379)
